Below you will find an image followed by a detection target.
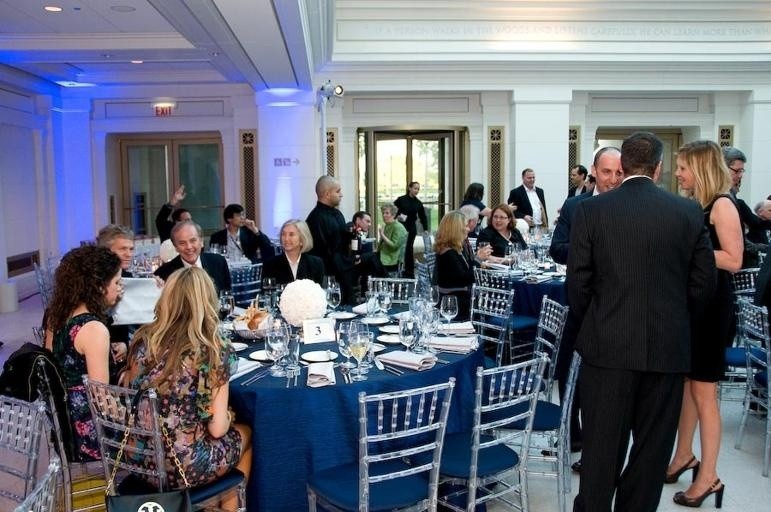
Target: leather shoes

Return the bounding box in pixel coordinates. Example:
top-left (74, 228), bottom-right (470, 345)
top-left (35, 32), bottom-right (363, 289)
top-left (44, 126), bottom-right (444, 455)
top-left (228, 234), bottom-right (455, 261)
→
top-left (541, 440), bottom-right (582, 456)
top-left (572, 459), bottom-right (581, 470)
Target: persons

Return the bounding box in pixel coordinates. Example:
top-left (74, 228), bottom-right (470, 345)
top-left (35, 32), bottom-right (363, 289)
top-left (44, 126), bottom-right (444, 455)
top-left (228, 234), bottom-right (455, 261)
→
top-left (209, 204), bottom-right (270, 264)
top-left (699, 146), bottom-right (771, 269)
top-left (155, 220), bottom-right (232, 298)
top-left (359, 203), bottom-right (408, 297)
top-left (476, 203), bottom-right (529, 264)
top-left (661, 140), bottom-right (746, 509)
top-left (541, 146), bottom-right (628, 475)
top-left (743, 254), bottom-right (771, 418)
top-left (156, 184), bottom-right (192, 244)
top-left (746, 199), bottom-right (771, 244)
top-left (305, 175), bottom-right (361, 306)
top-left (554, 164), bottom-right (588, 225)
top-left (393, 181), bottom-right (429, 284)
top-left (260, 219), bottom-right (324, 311)
top-left (460, 204), bottom-right (480, 265)
top-left (584, 175), bottom-right (596, 192)
top-left (43, 244), bottom-right (128, 461)
top-left (95, 225), bottom-right (167, 341)
top-left (507, 168), bottom-right (549, 233)
top-left (565, 130), bottom-right (718, 511)
top-left (347, 211), bottom-right (373, 254)
top-left (431, 211), bottom-right (494, 322)
top-left (459, 182), bottom-right (517, 238)
top-left (121, 266), bottom-right (254, 512)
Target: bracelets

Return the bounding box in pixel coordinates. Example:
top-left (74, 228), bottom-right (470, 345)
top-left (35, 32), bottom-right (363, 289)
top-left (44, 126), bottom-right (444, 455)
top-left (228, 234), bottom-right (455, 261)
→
top-left (227, 410), bottom-right (234, 423)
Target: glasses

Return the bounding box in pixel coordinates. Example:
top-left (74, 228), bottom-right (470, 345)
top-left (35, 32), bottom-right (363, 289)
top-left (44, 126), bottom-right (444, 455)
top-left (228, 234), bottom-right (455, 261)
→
top-left (729, 166), bottom-right (745, 174)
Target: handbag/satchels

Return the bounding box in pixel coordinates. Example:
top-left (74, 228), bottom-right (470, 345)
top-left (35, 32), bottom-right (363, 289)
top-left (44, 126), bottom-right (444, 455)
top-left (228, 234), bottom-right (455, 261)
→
top-left (104, 488), bottom-right (192, 512)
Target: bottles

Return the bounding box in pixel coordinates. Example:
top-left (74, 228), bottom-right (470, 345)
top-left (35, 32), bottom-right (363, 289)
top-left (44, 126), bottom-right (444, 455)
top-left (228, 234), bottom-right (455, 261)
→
top-left (348, 223), bottom-right (361, 262)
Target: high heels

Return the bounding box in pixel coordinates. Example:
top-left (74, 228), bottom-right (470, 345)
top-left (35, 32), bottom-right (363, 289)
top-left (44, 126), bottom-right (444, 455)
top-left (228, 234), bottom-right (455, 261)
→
top-left (672, 478), bottom-right (724, 508)
top-left (664, 455), bottom-right (700, 484)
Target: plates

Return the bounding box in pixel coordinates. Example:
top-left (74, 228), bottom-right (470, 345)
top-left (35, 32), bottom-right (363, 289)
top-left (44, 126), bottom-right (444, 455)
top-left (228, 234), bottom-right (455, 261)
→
top-left (543, 272), bottom-right (563, 277)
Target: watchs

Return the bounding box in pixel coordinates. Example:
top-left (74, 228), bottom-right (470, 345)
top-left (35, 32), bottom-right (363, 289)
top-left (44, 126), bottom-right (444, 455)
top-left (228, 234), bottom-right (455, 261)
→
top-left (167, 201), bottom-right (176, 207)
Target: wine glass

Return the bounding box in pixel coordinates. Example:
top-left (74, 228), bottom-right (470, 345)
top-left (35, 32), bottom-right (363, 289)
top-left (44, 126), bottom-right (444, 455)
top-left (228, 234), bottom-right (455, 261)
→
top-left (477, 225), bottom-right (552, 279)
top-left (217, 274), bottom-right (459, 382)
top-left (209, 243), bottom-right (242, 266)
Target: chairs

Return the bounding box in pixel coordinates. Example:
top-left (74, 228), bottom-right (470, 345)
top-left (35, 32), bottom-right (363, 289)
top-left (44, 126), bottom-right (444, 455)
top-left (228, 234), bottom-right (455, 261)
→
top-left (714, 235), bottom-right (769, 478)
top-left (0, 233), bottom-right (583, 512)
